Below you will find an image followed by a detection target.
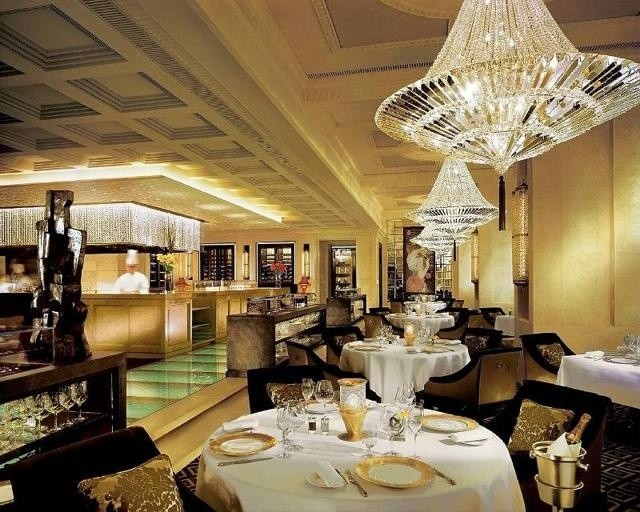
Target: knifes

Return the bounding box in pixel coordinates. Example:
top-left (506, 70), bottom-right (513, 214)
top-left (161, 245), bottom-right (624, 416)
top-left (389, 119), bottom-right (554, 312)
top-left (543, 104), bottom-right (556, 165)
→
top-left (345, 469), bottom-right (368, 498)
top-left (217, 457), bottom-right (273, 467)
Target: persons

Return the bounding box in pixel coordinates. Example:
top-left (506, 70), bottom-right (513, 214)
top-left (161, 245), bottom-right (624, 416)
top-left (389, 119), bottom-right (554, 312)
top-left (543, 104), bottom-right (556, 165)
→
top-left (112, 264), bottom-right (149, 293)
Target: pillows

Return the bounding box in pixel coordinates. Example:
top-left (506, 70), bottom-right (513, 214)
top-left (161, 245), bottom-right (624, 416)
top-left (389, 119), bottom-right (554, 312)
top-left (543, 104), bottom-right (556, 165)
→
top-left (507, 398), bottom-right (575, 453)
top-left (537, 342), bottom-right (567, 367)
top-left (75, 454), bottom-right (184, 510)
top-left (268, 381), bottom-right (321, 407)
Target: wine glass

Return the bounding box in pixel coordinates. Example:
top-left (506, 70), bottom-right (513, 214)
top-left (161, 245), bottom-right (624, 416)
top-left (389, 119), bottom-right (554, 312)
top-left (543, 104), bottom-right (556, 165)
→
top-left (407, 402), bottom-right (424, 443)
top-left (1, 381), bottom-right (88, 455)
top-left (301, 378), bottom-right (334, 416)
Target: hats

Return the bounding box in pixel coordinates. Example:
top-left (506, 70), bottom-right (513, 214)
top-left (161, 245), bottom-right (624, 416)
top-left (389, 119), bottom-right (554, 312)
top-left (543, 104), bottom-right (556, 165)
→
top-left (125, 249), bottom-right (139, 265)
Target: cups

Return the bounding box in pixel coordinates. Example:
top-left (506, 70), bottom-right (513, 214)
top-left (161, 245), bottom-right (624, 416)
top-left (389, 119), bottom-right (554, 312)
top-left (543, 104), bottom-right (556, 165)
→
top-left (276, 400), bottom-right (304, 458)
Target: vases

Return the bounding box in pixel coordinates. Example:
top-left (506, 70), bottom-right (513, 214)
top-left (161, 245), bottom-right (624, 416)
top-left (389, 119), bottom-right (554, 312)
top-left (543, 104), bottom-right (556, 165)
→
top-left (275, 270), bottom-right (282, 288)
top-left (164, 272), bottom-right (172, 294)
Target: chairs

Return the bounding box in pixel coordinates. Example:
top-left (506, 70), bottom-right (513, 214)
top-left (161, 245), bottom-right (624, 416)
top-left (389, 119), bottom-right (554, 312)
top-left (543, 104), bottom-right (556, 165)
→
top-left (519, 331), bottom-right (577, 385)
top-left (246, 364), bottom-right (325, 415)
top-left (5, 288), bottom-right (65, 371)
top-left (495, 379), bottom-right (611, 510)
top-left (247, 287), bottom-right (509, 382)
top-left (3, 425), bottom-right (214, 509)
top-left (416, 345), bottom-right (503, 416)
top-left (306, 348), bottom-right (372, 399)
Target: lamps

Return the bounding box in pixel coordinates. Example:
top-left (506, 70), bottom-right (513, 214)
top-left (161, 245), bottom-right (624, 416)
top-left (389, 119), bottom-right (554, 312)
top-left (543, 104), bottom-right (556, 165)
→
top-left (402, 155), bottom-right (508, 264)
top-left (409, 223), bottom-right (470, 268)
top-left (298, 277), bottom-right (311, 294)
top-left (375, 3), bottom-right (640, 232)
top-left (175, 277), bottom-right (189, 290)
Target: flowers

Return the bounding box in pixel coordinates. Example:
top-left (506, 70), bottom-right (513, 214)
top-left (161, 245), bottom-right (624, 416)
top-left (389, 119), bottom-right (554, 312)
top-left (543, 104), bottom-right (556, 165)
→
top-left (270, 261), bottom-right (286, 273)
top-left (156, 253), bottom-right (184, 274)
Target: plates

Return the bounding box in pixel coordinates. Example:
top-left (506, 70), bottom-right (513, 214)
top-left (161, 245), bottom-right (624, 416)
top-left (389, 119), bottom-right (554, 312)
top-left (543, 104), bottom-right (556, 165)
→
top-left (420, 416), bottom-right (478, 432)
top-left (355, 456), bottom-right (436, 488)
top-left (209, 432), bottom-right (277, 457)
top-left (306, 471), bottom-right (348, 488)
top-left (353, 345), bottom-right (380, 351)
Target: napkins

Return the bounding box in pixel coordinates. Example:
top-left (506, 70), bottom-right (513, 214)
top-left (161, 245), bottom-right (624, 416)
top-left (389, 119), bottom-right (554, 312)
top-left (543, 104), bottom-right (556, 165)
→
top-left (221, 418), bottom-right (259, 432)
top-left (314, 459), bottom-right (344, 490)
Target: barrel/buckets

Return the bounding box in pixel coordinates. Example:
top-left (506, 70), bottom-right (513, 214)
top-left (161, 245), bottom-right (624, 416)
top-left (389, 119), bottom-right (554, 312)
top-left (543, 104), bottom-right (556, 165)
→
top-left (529, 439), bottom-right (590, 507)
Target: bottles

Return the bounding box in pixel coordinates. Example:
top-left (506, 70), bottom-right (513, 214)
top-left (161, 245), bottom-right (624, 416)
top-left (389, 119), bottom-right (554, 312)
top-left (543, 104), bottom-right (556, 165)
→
top-left (567, 413), bottom-right (592, 444)
top-left (308, 417), bottom-right (329, 435)
